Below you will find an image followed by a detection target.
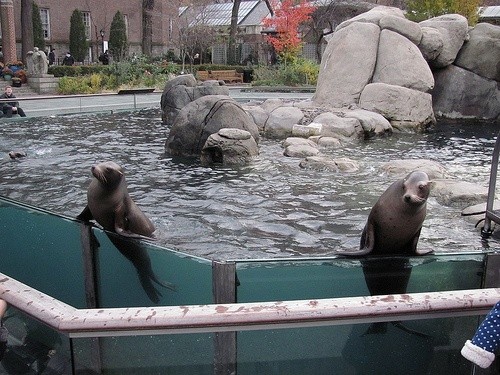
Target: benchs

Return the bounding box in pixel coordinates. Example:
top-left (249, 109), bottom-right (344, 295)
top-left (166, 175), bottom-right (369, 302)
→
top-left (12, 93), bottom-right (316, 117)
top-left (196, 69), bottom-right (244, 84)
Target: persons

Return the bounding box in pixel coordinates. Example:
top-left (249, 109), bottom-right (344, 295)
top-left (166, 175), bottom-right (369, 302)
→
top-left (102, 49), bottom-right (109, 65)
top-left (62, 51), bottom-right (75, 66)
top-left (0, 86), bottom-right (27, 118)
top-left (48, 48), bottom-right (55, 67)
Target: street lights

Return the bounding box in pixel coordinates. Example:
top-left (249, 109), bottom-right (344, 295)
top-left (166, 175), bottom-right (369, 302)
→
top-left (100, 30), bottom-right (105, 58)
